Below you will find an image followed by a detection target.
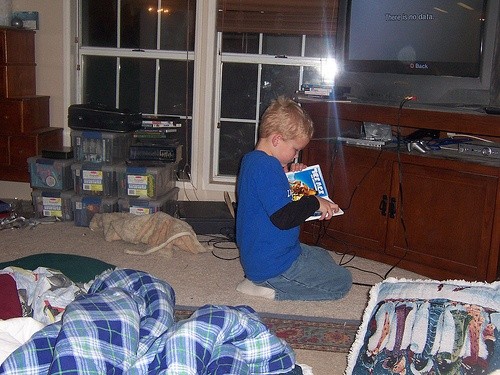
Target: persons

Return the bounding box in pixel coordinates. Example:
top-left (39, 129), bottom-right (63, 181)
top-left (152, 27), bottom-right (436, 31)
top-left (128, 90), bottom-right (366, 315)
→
top-left (235, 96), bottom-right (353, 301)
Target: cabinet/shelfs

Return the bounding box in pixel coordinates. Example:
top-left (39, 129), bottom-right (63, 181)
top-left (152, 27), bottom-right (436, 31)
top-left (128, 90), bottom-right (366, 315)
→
top-left (293, 96), bottom-right (500, 283)
top-left (0, 25), bottom-right (63, 183)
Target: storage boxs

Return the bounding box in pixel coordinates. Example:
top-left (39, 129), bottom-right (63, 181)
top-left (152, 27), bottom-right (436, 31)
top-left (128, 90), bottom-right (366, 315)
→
top-left (12, 11), bottom-right (39, 31)
top-left (26, 104), bottom-right (236, 234)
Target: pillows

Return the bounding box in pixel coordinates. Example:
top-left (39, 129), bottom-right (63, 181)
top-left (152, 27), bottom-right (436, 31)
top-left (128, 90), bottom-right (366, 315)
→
top-left (346, 276), bottom-right (500, 375)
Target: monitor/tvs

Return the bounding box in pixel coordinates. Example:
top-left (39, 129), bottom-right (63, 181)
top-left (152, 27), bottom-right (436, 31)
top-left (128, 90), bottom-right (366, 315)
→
top-left (336, 0), bottom-right (500, 105)
